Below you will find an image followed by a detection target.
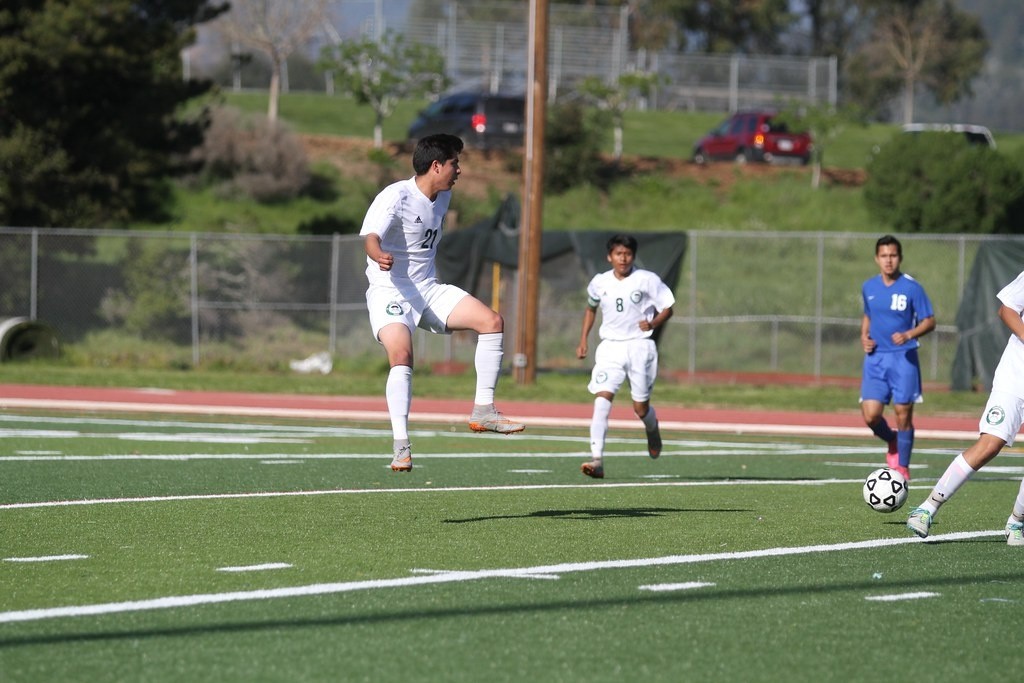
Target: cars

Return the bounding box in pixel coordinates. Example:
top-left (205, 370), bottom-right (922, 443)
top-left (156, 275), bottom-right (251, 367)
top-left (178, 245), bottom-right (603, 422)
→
top-left (401, 82), bottom-right (526, 157)
top-left (889, 121), bottom-right (998, 181)
top-left (690, 111), bottom-right (812, 169)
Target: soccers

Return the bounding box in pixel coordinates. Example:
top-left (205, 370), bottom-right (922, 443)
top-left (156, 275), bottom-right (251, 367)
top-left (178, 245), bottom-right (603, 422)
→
top-left (862, 467), bottom-right (908, 513)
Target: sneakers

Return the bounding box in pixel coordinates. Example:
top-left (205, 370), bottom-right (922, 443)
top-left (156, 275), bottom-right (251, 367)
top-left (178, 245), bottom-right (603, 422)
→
top-left (645, 419), bottom-right (661, 458)
top-left (908, 507), bottom-right (933, 538)
top-left (470, 411), bottom-right (526, 435)
top-left (886, 431), bottom-right (899, 470)
top-left (1005, 519), bottom-right (1024, 547)
top-left (900, 466), bottom-right (910, 481)
top-left (582, 458), bottom-right (603, 479)
top-left (391, 439), bottom-right (412, 472)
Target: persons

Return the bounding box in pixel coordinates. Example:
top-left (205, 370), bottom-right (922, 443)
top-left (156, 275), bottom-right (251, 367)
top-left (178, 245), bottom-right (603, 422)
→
top-left (577, 234), bottom-right (676, 480)
top-left (907, 269), bottom-right (1024, 546)
top-left (359, 134), bottom-right (526, 473)
top-left (859, 235), bottom-right (937, 479)
